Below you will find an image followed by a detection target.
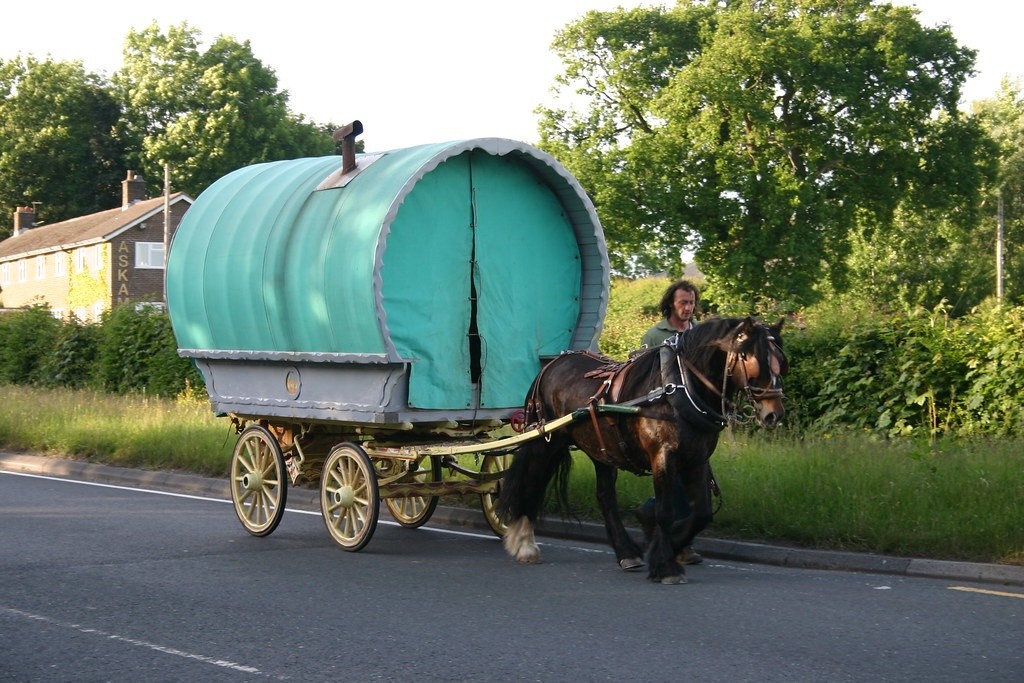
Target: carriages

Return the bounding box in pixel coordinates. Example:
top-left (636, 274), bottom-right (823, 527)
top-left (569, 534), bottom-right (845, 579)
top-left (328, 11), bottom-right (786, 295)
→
top-left (164, 122), bottom-right (787, 586)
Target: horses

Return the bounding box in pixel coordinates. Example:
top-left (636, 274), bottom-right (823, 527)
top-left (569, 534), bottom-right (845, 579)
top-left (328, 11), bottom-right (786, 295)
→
top-left (491, 316), bottom-right (789, 585)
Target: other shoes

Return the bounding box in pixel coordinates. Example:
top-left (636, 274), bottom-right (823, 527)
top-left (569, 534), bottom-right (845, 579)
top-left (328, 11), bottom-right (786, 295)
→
top-left (637, 506), bottom-right (655, 544)
top-left (676, 544), bottom-right (703, 566)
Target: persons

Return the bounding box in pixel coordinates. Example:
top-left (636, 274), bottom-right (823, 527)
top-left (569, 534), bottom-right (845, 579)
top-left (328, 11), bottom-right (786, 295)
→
top-left (638, 280), bottom-right (709, 564)
